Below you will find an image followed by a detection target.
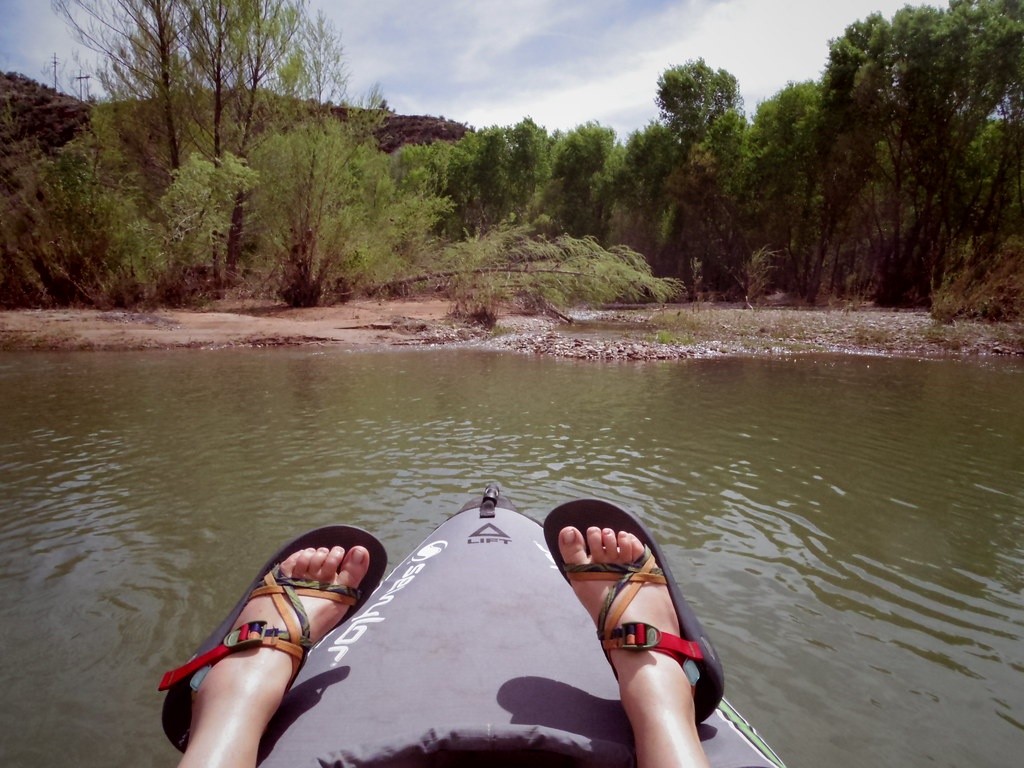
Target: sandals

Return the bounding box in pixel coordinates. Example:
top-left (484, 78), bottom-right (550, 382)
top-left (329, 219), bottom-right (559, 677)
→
top-left (157, 523), bottom-right (388, 755)
top-left (544, 497), bottom-right (725, 726)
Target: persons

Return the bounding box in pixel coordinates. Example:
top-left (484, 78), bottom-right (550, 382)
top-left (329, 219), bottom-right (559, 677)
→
top-left (158, 497), bottom-right (727, 767)
